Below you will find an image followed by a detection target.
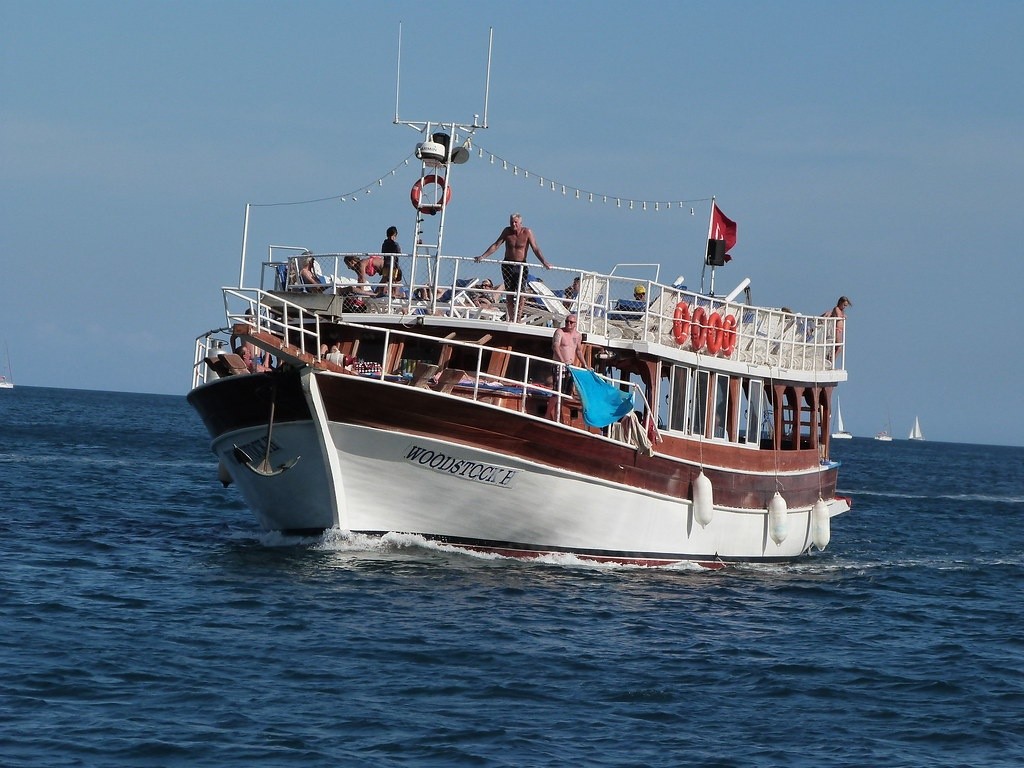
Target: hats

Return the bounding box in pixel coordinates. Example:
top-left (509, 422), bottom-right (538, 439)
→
top-left (634, 284), bottom-right (646, 293)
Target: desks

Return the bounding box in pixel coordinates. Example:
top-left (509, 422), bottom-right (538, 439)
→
top-left (425, 381), bottom-right (532, 410)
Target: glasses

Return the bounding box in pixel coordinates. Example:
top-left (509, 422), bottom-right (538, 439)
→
top-left (565, 320), bottom-right (576, 324)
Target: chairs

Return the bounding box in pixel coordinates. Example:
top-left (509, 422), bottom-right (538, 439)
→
top-left (276, 265), bottom-right (837, 369)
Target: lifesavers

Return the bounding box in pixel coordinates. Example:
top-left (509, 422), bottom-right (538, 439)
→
top-left (410, 174), bottom-right (451, 214)
top-left (707, 312), bottom-right (724, 354)
top-left (691, 307), bottom-right (708, 349)
top-left (723, 314), bottom-right (736, 356)
top-left (673, 301), bottom-right (690, 344)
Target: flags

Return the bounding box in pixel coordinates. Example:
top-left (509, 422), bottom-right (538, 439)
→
top-left (711, 203), bottom-right (737, 254)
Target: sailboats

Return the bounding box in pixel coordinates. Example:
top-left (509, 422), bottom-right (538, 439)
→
top-left (908, 416), bottom-right (925, 442)
top-left (0, 339), bottom-right (13, 389)
top-left (831, 394), bottom-right (852, 439)
top-left (874, 404), bottom-right (892, 441)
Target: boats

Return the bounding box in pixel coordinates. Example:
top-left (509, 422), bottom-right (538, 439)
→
top-left (184, 32), bottom-right (852, 565)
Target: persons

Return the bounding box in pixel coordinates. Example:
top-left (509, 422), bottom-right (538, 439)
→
top-left (474, 212), bottom-right (551, 323)
top-left (331, 345), bottom-right (338, 353)
top-left (453, 278), bottom-right (505, 310)
top-left (230, 309), bottom-right (269, 368)
top-left (634, 285), bottom-right (645, 301)
top-left (544, 315), bottom-right (593, 420)
top-left (827, 296), bottom-right (851, 363)
top-left (562, 277), bottom-right (580, 311)
top-left (320, 344), bottom-right (328, 359)
top-left (343, 255), bottom-right (384, 291)
top-left (300, 252), bottom-right (379, 297)
top-left (381, 226), bottom-right (402, 298)
